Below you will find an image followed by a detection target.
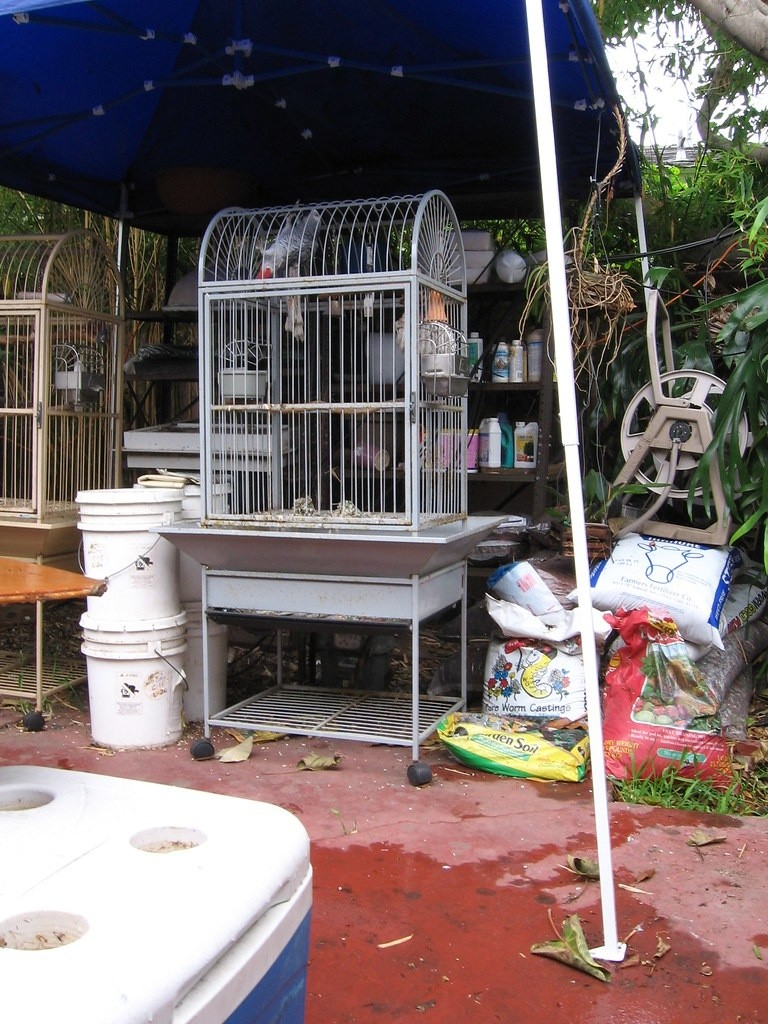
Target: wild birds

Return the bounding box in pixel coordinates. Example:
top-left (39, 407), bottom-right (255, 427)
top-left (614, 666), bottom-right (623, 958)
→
top-left (251, 199), bottom-right (321, 285)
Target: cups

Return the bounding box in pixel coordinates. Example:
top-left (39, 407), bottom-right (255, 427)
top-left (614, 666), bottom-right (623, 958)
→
top-left (355, 443), bottom-right (389, 470)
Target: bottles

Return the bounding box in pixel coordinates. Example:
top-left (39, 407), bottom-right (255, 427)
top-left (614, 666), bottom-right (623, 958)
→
top-left (468, 332), bottom-right (483, 382)
top-left (527, 323), bottom-right (542, 382)
top-left (514, 421), bottom-right (538, 468)
top-left (509, 340), bottom-right (523, 382)
top-left (478, 417), bottom-right (501, 467)
top-left (493, 337), bottom-right (508, 383)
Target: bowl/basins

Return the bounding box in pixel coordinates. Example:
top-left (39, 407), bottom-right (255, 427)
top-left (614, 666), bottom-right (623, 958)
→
top-left (220, 371), bottom-right (267, 399)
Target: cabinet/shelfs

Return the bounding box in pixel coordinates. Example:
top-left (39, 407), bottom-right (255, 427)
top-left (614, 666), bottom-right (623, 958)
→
top-left (125, 281), bottom-right (555, 761)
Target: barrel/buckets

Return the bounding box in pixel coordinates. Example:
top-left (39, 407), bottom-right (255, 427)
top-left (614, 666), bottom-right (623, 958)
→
top-left (74, 489), bottom-right (185, 620)
top-left (133, 485), bottom-right (231, 604)
top-left (180, 600), bottom-right (228, 723)
top-left (79, 611), bottom-right (189, 749)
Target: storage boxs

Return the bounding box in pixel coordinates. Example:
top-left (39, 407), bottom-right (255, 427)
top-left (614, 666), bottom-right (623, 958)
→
top-left (446, 232), bottom-right (496, 285)
top-left (0, 764), bottom-right (313, 1024)
